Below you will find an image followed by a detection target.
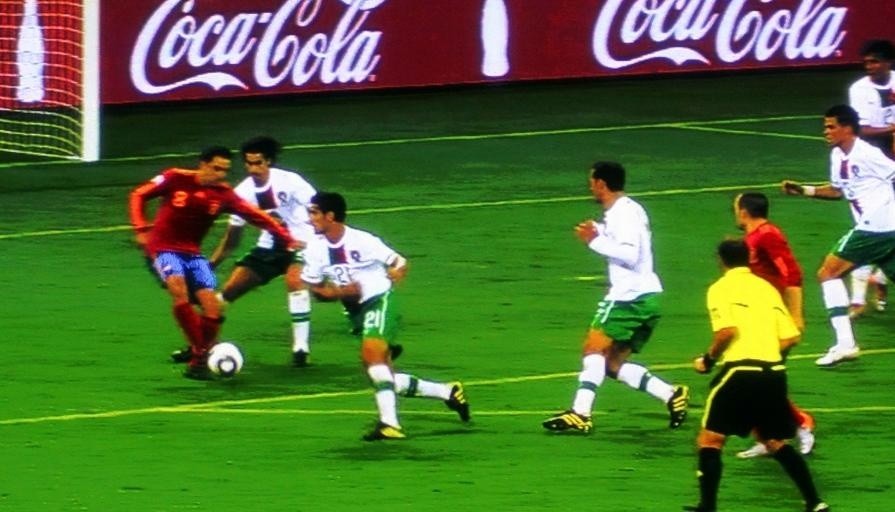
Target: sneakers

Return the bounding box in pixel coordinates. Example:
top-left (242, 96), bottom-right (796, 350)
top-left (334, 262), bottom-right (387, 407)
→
top-left (668, 384), bottom-right (689, 428)
top-left (814, 344), bottom-right (861, 367)
top-left (737, 443), bottom-right (770, 459)
top-left (289, 352), bottom-right (309, 365)
top-left (797, 414), bottom-right (815, 454)
top-left (542, 409), bottom-right (591, 434)
top-left (172, 345), bottom-right (214, 380)
top-left (444, 380), bottom-right (468, 420)
top-left (364, 422), bottom-right (405, 439)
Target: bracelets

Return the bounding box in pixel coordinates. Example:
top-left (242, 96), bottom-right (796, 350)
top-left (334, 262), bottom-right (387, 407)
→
top-left (802, 185), bottom-right (816, 198)
top-left (703, 353), bottom-right (714, 369)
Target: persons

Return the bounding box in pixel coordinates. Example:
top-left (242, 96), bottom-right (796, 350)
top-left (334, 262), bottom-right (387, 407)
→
top-left (848, 38), bottom-right (895, 321)
top-left (128, 144), bottom-right (308, 381)
top-left (683, 239), bottom-right (828, 510)
top-left (300, 189), bottom-right (471, 441)
top-left (780, 105), bottom-right (894, 365)
top-left (169, 134), bottom-right (317, 365)
top-left (732, 192), bottom-right (816, 461)
top-left (542, 159), bottom-right (691, 433)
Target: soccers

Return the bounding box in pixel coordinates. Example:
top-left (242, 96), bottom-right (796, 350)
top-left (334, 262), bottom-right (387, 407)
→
top-left (208, 343), bottom-right (244, 376)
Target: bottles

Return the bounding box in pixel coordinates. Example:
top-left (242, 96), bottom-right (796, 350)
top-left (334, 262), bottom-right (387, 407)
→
top-left (479, 2), bottom-right (513, 77)
top-left (13, 0), bottom-right (48, 103)
